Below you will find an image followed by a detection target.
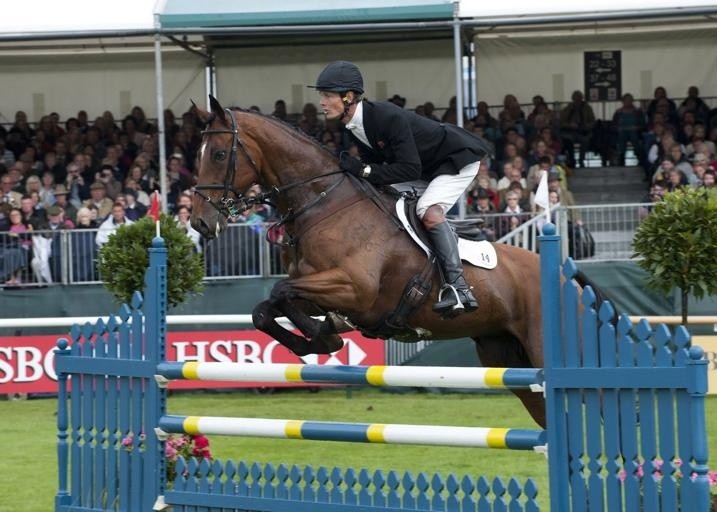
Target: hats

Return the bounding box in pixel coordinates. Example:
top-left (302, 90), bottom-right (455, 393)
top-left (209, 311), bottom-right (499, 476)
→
top-left (90, 182), bottom-right (104, 189)
top-left (51, 183), bottom-right (71, 196)
top-left (46, 206), bottom-right (61, 216)
top-left (473, 188), bottom-right (493, 199)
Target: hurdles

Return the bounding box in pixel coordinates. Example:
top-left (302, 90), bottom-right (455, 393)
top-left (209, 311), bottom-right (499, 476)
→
top-left (53, 222), bottom-right (709, 512)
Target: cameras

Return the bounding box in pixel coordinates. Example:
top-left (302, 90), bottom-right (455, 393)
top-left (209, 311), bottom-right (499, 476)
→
top-left (72, 174), bottom-right (78, 180)
top-left (100, 173), bottom-right (106, 178)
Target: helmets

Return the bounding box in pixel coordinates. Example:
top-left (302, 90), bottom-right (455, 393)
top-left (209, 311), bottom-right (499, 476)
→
top-left (307, 61), bottom-right (364, 94)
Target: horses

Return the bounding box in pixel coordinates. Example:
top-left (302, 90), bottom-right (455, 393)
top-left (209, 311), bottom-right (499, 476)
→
top-left (189, 93), bottom-right (640, 511)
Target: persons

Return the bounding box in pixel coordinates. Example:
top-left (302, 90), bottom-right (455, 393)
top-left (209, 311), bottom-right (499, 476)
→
top-left (467, 91), bottom-right (595, 255)
top-left (612, 87), bottom-right (716, 221)
top-left (272, 100), bottom-right (362, 160)
top-left (305, 61), bottom-right (489, 318)
top-left (386, 94), bottom-right (469, 125)
top-left (0, 107), bottom-right (277, 276)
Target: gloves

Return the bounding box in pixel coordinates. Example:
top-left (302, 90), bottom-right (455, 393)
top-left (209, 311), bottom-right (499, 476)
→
top-left (339, 153), bottom-right (362, 177)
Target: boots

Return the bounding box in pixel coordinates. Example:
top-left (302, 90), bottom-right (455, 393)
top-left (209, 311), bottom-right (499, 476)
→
top-left (422, 204), bottom-right (479, 313)
